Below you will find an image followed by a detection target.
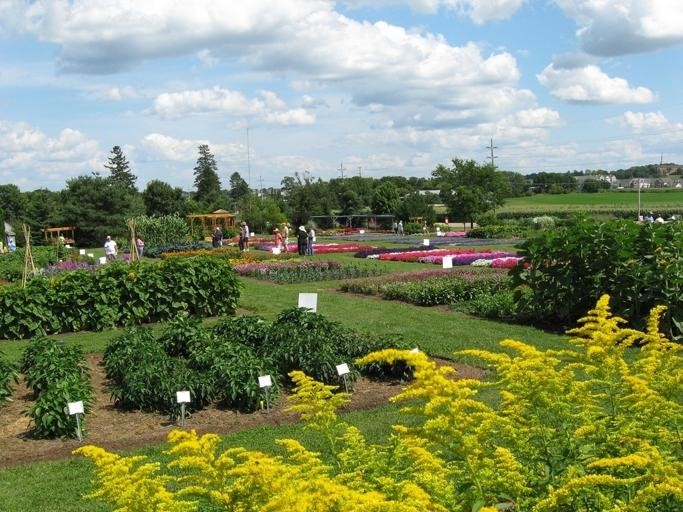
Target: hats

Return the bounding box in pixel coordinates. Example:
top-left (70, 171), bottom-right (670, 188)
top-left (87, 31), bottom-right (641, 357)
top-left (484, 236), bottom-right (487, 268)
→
top-left (240, 222), bottom-right (246, 226)
top-left (106, 235), bottom-right (111, 239)
top-left (273, 228), bottom-right (280, 231)
top-left (281, 223), bottom-right (286, 225)
top-left (216, 227), bottom-right (220, 231)
top-left (299, 226), bottom-right (305, 231)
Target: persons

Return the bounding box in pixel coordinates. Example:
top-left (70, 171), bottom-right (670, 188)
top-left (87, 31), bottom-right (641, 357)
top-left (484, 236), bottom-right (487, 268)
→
top-left (295, 225), bottom-right (308, 257)
top-left (272, 228), bottom-right (282, 249)
top-left (392, 220), bottom-right (399, 237)
top-left (240, 221), bottom-right (249, 253)
top-left (238, 226), bottom-right (245, 252)
top-left (281, 223), bottom-right (289, 253)
top-left (6, 238), bottom-right (16, 252)
top-left (135, 234), bottom-right (145, 257)
top-left (211, 229), bottom-right (217, 248)
top-left (654, 213), bottom-right (664, 223)
top-left (103, 235), bottom-right (118, 262)
top-left (396, 220), bottom-right (404, 238)
top-left (213, 227), bottom-right (223, 248)
top-left (642, 210), bottom-right (654, 223)
top-left (305, 225), bottom-right (316, 256)
